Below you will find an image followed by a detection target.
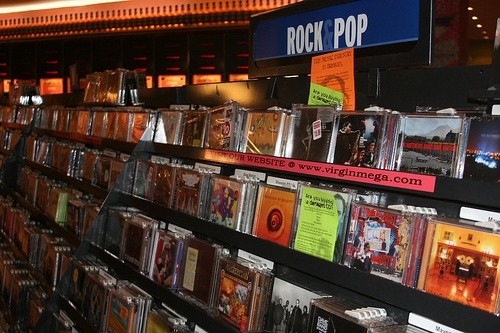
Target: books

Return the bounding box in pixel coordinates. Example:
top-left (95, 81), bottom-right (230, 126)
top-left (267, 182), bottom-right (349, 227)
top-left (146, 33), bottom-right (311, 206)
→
top-left (0, 28), bottom-right (499, 332)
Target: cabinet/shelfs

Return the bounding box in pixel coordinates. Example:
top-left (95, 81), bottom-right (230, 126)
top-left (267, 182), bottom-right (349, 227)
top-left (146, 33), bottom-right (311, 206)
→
top-left (0, 65), bottom-right (500, 332)
